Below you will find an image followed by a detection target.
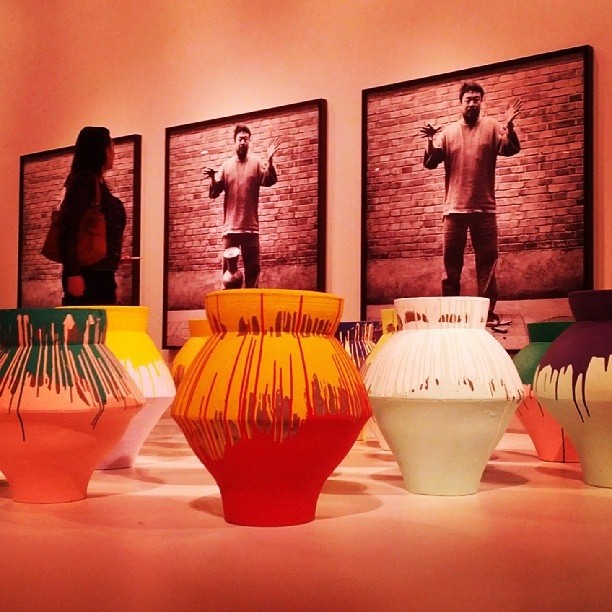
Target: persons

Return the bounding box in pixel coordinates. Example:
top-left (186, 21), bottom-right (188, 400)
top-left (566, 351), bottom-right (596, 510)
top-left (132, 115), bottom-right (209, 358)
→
top-left (200, 125), bottom-right (282, 287)
top-left (420, 82), bottom-right (523, 323)
top-left (41, 127), bottom-right (126, 305)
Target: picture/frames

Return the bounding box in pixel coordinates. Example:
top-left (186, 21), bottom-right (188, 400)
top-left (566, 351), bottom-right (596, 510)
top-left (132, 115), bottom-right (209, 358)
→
top-left (360, 45), bottom-right (594, 322)
top-left (17, 134), bottom-right (140, 306)
top-left (163, 99), bottom-right (328, 348)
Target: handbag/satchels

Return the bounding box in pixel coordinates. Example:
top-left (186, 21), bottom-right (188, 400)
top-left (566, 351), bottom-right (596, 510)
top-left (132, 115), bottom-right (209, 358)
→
top-left (40, 208), bottom-right (107, 265)
